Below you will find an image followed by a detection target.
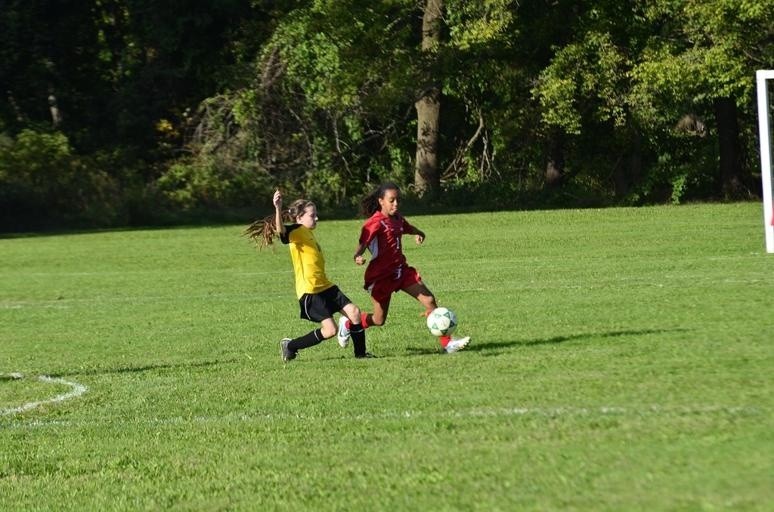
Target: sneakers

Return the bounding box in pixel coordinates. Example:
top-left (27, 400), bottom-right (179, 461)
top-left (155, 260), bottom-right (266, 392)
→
top-left (338, 316), bottom-right (351, 348)
top-left (444, 337), bottom-right (471, 354)
top-left (360, 353), bottom-right (377, 358)
top-left (280, 338), bottom-right (298, 363)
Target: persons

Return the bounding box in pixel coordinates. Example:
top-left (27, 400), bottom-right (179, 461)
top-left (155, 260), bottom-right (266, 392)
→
top-left (337, 180), bottom-right (472, 355)
top-left (242, 188), bottom-right (378, 361)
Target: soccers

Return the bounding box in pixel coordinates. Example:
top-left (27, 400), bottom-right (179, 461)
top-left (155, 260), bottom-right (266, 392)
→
top-left (427, 306), bottom-right (457, 336)
